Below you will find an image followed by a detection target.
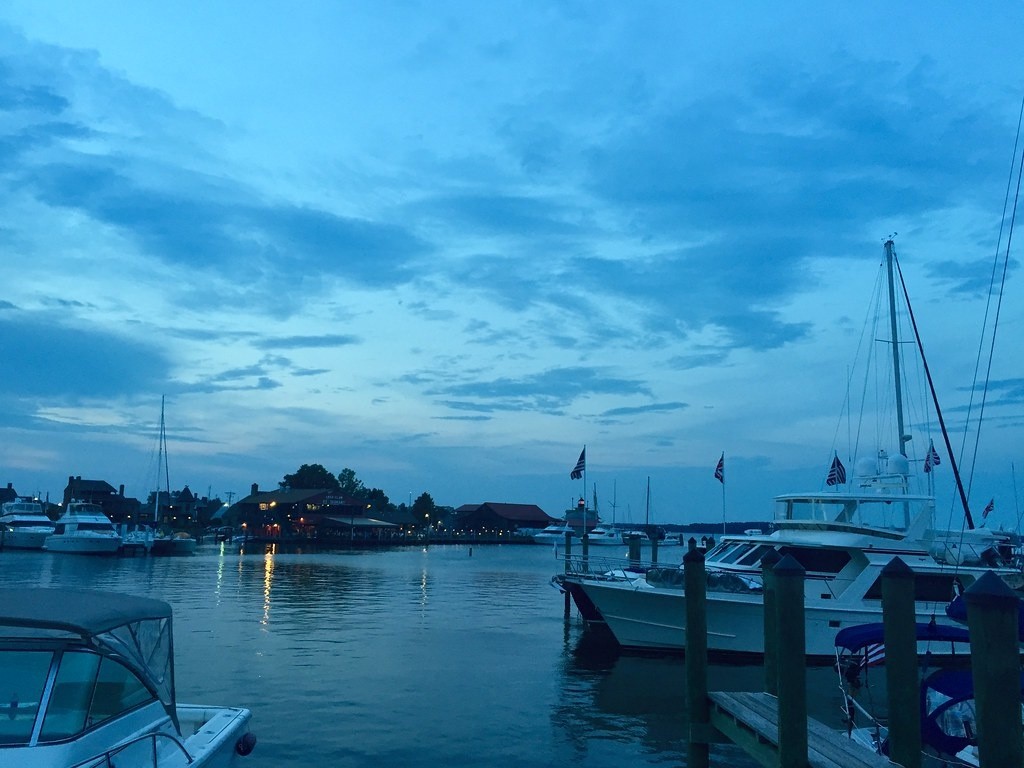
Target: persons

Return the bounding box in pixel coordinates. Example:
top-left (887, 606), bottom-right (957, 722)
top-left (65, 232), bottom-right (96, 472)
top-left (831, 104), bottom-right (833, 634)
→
top-left (951, 575), bottom-right (965, 602)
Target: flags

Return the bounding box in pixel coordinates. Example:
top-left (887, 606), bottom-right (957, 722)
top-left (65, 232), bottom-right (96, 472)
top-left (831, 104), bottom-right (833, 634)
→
top-left (715, 453), bottom-right (725, 484)
top-left (924, 438), bottom-right (940, 473)
top-left (981, 496), bottom-right (995, 518)
top-left (826, 455), bottom-right (846, 486)
top-left (570, 445), bottom-right (585, 480)
